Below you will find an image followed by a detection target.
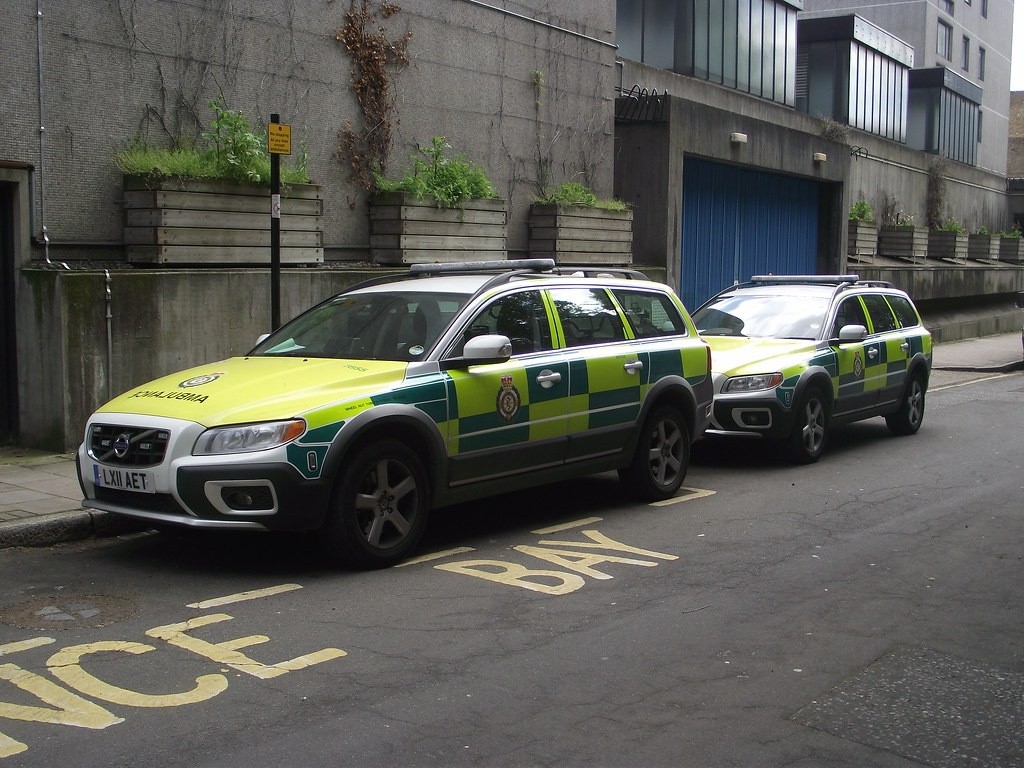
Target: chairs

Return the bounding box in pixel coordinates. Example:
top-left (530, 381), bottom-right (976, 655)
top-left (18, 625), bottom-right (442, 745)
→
top-left (497, 301), bottom-right (535, 355)
top-left (400, 299), bottom-right (444, 358)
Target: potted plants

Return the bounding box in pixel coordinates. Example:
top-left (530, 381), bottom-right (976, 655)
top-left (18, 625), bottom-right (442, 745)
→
top-left (367, 133), bottom-right (511, 270)
top-left (847, 201), bottom-right (878, 264)
top-left (881, 217), bottom-right (1024, 265)
top-left (113, 94), bottom-right (326, 267)
top-left (527, 171), bottom-right (635, 267)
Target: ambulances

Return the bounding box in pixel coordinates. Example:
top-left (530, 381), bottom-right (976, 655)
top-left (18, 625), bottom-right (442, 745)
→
top-left (75, 258), bottom-right (714, 569)
top-left (662, 273), bottom-right (933, 466)
